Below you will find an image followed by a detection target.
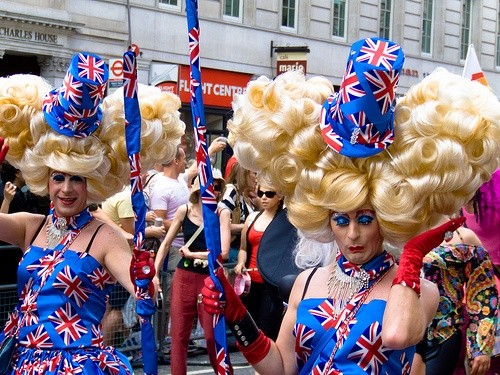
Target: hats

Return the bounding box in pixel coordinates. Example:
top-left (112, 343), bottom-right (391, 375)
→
top-left (43, 52), bottom-right (110, 138)
top-left (319, 37), bottom-right (406, 157)
top-left (190, 170), bottom-right (227, 203)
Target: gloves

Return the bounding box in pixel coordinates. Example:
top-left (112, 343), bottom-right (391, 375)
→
top-left (200, 267), bottom-right (270, 365)
top-left (129, 247), bottom-right (156, 300)
top-left (391, 217), bottom-right (467, 296)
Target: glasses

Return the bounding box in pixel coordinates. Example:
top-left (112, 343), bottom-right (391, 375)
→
top-left (214, 184), bottom-right (222, 192)
top-left (257, 189), bottom-right (278, 198)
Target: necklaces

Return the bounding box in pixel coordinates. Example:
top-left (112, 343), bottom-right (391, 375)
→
top-left (328, 263), bottom-right (366, 321)
top-left (46, 221), bottom-right (69, 249)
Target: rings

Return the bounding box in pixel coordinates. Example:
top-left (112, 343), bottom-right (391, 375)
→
top-left (142, 264), bottom-right (151, 276)
top-left (444, 231), bottom-right (454, 241)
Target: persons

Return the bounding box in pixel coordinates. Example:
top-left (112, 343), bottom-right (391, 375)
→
top-left (0, 143), bottom-right (158, 375)
top-left (97, 148), bottom-right (281, 362)
top-left (234, 181), bottom-right (288, 343)
top-left (417, 227), bottom-right (500, 375)
top-left (150, 168), bottom-right (234, 374)
top-left (200, 165), bottom-right (442, 375)
top-left (461, 186), bottom-right (500, 275)
top-left (1, 165), bottom-right (28, 213)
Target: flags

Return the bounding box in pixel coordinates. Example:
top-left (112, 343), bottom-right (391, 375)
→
top-left (463, 43), bottom-right (491, 86)
